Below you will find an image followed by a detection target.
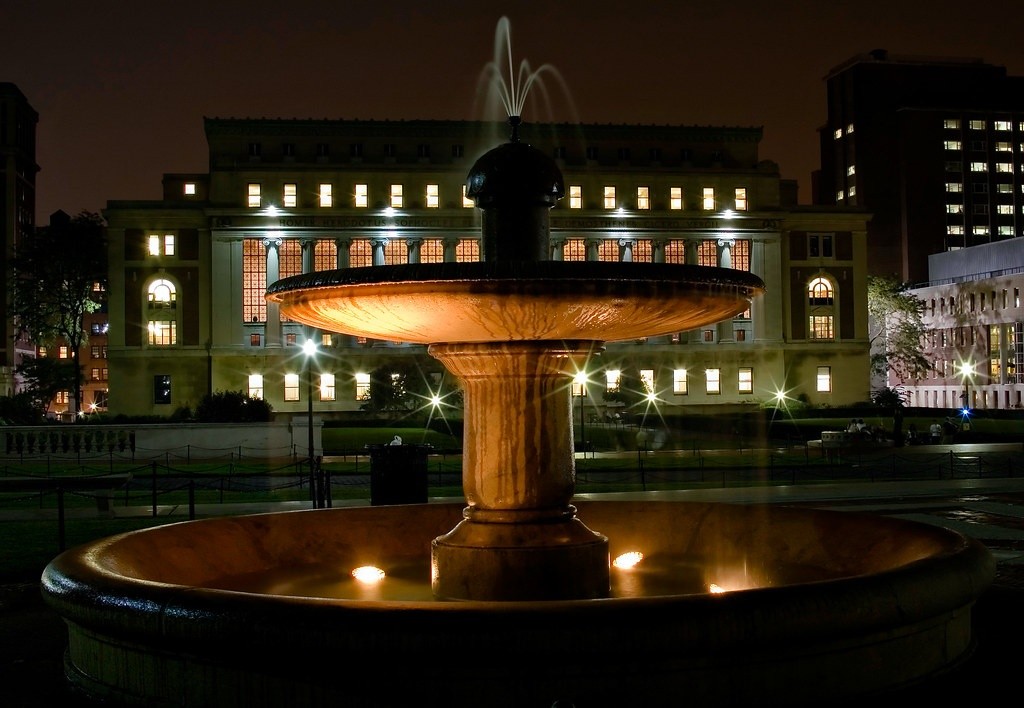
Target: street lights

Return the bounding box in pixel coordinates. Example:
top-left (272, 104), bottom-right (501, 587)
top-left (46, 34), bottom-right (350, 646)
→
top-left (303, 339), bottom-right (318, 501)
top-left (962, 362), bottom-right (972, 411)
top-left (578, 374), bottom-right (585, 452)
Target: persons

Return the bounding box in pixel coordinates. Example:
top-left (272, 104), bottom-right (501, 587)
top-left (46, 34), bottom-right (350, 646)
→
top-left (846, 415), bottom-right (959, 446)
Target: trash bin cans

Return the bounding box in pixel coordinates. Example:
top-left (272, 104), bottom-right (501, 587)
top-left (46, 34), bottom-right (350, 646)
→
top-left (290, 416), bottom-right (324, 457)
top-left (364, 442), bottom-right (435, 506)
